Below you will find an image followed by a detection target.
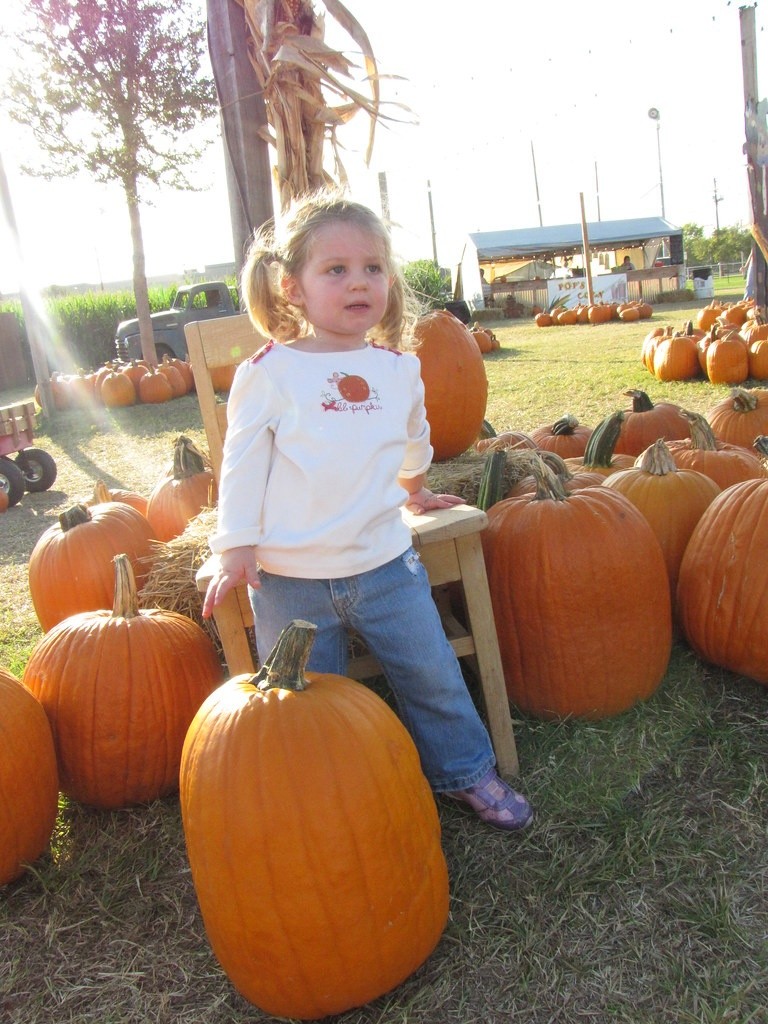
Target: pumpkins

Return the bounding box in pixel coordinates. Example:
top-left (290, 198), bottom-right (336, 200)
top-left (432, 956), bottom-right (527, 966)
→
top-left (0, 298), bottom-right (768, 1024)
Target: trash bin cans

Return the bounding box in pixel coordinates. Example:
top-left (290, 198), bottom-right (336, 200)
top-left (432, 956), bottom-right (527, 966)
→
top-left (692, 268), bottom-right (714, 299)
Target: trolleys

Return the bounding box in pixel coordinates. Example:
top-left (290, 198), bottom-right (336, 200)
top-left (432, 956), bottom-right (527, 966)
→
top-left (0, 400), bottom-right (57, 512)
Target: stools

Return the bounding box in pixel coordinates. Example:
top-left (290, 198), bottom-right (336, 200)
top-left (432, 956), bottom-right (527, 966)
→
top-left (179, 313), bottom-right (520, 776)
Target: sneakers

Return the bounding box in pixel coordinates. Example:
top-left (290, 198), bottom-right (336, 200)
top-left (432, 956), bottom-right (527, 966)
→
top-left (438, 764), bottom-right (534, 833)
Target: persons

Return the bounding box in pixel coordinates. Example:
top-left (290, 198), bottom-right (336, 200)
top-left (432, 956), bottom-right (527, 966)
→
top-left (201, 198), bottom-right (535, 832)
top-left (623, 256), bottom-right (636, 270)
top-left (739, 252), bottom-right (754, 300)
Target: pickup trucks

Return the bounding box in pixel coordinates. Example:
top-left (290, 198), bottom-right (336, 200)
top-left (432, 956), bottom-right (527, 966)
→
top-left (115, 281), bottom-right (244, 364)
